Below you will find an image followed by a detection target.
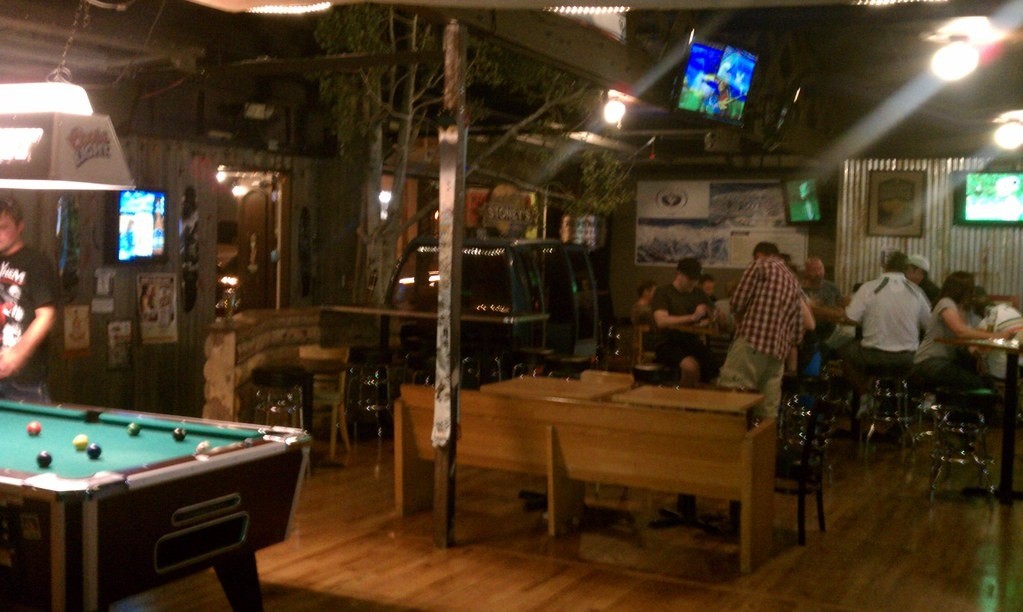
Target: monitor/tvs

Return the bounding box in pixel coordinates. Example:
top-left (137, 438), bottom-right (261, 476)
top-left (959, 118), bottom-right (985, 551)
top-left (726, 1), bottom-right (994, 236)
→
top-left (953, 170), bottom-right (1023, 228)
top-left (673, 38), bottom-right (759, 129)
top-left (117, 188), bottom-right (185, 262)
top-left (781, 172), bottom-right (827, 225)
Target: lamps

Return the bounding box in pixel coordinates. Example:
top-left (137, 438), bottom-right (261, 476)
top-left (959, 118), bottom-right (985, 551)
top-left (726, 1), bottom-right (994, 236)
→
top-left (0, 0), bottom-right (136, 190)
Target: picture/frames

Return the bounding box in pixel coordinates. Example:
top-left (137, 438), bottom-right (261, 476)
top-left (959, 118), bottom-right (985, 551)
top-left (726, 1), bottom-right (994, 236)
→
top-left (867, 170), bottom-right (926, 238)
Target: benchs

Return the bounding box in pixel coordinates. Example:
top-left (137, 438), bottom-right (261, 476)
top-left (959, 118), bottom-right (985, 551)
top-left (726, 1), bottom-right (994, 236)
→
top-left (394, 383), bottom-right (777, 571)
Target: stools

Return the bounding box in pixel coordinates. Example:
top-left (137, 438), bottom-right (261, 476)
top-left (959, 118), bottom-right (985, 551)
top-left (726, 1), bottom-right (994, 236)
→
top-left (242, 316), bottom-right (998, 535)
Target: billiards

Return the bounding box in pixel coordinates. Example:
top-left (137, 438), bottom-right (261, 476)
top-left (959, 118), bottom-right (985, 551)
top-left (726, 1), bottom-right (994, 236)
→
top-left (128, 423), bottom-right (140, 436)
top-left (87, 443), bottom-right (102, 458)
top-left (73, 434), bottom-right (88, 451)
top-left (27, 420), bottom-right (42, 435)
top-left (196, 441), bottom-right (211, 454)
top-left (37, 451), bottom-right (52, 467)
top-left (173, 427), bottom-right (187, 441)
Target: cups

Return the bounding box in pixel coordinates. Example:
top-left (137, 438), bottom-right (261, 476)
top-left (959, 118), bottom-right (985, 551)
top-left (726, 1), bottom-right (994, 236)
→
top-left (224, 290), bottom-right (236, 325)
top-left (987, 305), bottom-right (997, 334)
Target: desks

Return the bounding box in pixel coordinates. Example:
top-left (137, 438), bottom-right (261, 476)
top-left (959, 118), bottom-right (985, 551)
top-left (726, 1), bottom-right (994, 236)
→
top-left (0, 393), bottom-right (312, 612)
top-left (934, 338), bottom-right (1023, 504)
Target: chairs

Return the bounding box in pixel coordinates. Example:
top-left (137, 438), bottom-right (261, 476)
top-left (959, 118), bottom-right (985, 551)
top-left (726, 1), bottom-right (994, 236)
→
top-left (580, 371), bottom-right (634, 405)
top-left (728, 375), bottom-right (827, 545)
top-left (299, 342), bottom-right (356, 458)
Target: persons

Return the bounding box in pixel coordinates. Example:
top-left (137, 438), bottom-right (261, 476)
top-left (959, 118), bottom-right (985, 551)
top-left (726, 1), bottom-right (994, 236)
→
top-left (124, 219), bottom-right (136, 254)
top-left (839, 248), bottom-right (1023, 423)
top-left (0, 195), bottom-right (61, 404)
top-left (630, 239), bottom-right (846, 538)
top-left (141, 283), bottom-right (159, 318)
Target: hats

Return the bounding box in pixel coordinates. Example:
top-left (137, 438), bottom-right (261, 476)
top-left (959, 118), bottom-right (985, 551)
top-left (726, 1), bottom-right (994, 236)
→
top-left (906, 253), bottom-right (930, 272)
top-left (678, 258), bottom-right (703, 279)
